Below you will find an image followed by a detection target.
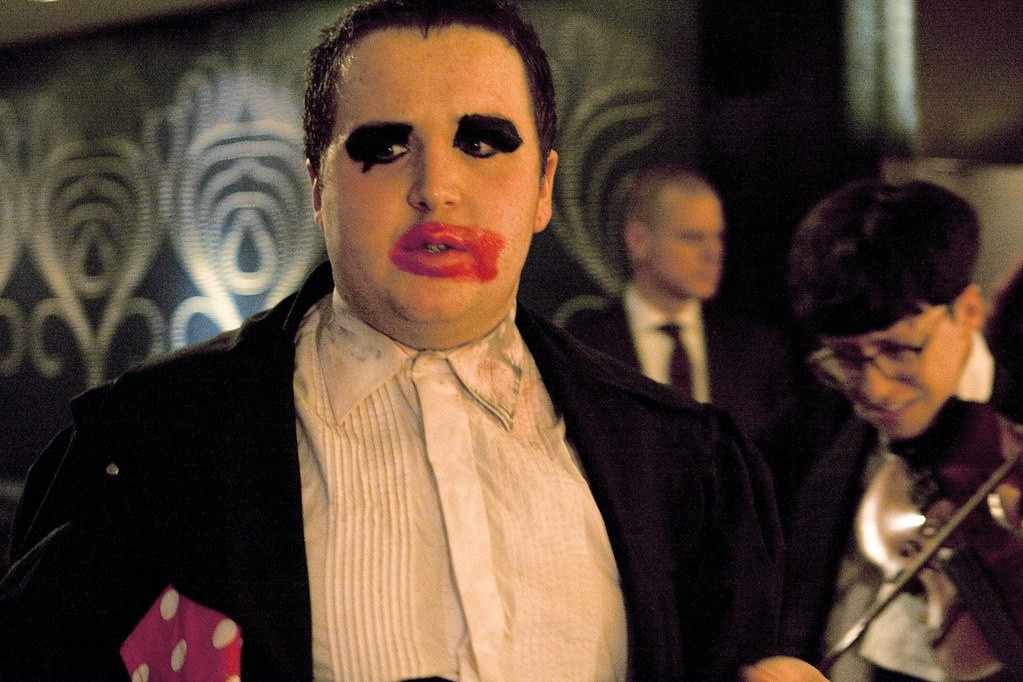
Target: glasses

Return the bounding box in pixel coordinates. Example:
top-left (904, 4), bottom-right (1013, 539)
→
top-left (808, 308), bottom-right (953, 390)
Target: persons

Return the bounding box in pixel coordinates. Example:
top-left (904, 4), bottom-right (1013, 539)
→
top-left (560, 163), bottom-right (795, 447)
top-left (776, 177), bottom-right (1023, 682)
top-left (0, 0), bottom-right (825, 681)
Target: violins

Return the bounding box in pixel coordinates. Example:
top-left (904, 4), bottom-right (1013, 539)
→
top-left (851, 396), bottom-right (1023, 682)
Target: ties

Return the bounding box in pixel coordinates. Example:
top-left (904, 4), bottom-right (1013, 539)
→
top-left (658, 321), bottom-right (692, 397)
